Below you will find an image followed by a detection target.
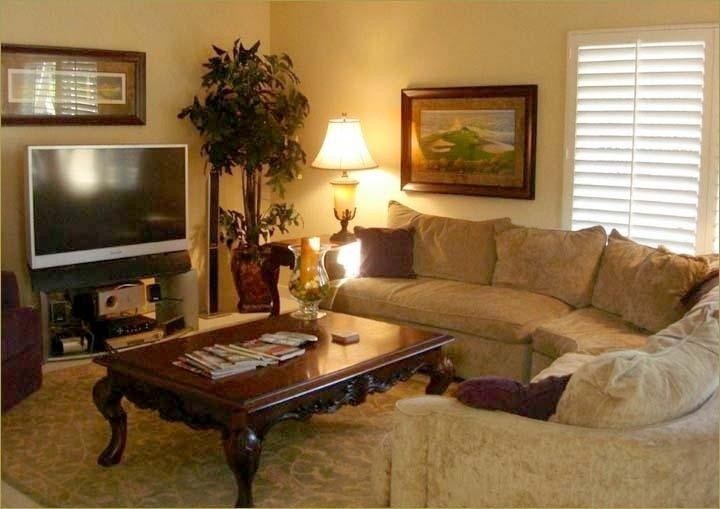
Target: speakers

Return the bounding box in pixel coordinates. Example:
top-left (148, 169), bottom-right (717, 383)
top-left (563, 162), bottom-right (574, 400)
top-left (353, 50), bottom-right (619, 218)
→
top-left (53, 302), bottom-right (66, 323)
top-left (147, 284), bottom-right (161, 303)
top-left (198, 168), bottom-right (231, 320)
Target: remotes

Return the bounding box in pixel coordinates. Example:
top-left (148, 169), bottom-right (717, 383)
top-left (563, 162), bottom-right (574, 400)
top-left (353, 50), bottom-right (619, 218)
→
top-left (261, 333), bottom-right (301, 347)
top-left (275, 331), bottom-right (318, 342)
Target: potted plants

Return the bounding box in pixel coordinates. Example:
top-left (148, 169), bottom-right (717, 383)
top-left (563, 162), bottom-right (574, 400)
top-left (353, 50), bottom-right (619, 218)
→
top-left (178, 40), bottom-right (312, 311)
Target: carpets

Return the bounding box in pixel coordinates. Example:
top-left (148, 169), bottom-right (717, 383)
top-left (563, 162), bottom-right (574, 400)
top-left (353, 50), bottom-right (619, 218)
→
top-left (1, 356), bottom-right (456, 508)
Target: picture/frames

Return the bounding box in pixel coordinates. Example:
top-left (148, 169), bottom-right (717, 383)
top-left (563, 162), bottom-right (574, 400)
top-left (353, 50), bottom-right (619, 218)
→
top-left (399, 84), bottom-right (538, 201)
top-left (2, 43), bottom-right (146, 128)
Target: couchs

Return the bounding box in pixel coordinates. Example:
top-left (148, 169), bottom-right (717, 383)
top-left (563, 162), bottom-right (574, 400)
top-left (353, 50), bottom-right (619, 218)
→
top-left (2, 270), bottom-right (42, 411)
top-left (323, 201), bottom-right (720, 509)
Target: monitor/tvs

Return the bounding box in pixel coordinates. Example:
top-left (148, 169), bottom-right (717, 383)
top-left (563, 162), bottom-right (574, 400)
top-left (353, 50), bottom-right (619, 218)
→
top-left (26, 143), bottom-right (189, 270)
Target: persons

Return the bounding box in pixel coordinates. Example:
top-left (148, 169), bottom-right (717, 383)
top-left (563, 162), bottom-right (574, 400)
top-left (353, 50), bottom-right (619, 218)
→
top-left (171, 330), bottom-right (319, 381)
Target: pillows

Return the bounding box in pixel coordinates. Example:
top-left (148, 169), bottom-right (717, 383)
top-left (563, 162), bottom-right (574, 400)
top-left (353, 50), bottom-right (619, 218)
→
top-left (354, 223), bottom-right (418, 281)
top-left (461, 371), bottom-right (570, 421)
top-left (544, 304), bottom-right (718, 431)
top-left (591, 228), bottom-right (656, 318)
top-left (490, 225), bottom-right (526, 288)
top-left (383, 200), bottom-right (511, 286)
top-left (620, 247), bottom-right (710, 335)
top-left (526, 224), bottom-right (608, 310)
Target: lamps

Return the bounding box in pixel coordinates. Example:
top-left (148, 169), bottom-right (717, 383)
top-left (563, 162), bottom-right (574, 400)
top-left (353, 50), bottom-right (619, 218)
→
top-left (311, 113), bottom-right (377, 244)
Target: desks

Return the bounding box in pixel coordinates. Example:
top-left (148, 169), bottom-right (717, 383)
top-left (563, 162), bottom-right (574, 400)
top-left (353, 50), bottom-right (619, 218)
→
top-left (267, 235), bottom-right (342, 317)
